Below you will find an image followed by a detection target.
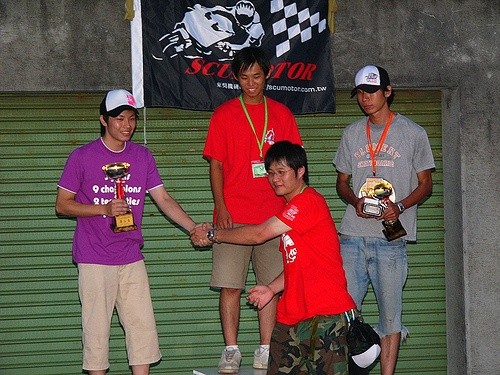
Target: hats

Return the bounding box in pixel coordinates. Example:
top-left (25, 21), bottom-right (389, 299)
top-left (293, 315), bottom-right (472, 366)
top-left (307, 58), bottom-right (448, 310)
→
top-left (100, 89), bottom-right (139, 118)
top-left (351, 65), bottom-right (390, 93)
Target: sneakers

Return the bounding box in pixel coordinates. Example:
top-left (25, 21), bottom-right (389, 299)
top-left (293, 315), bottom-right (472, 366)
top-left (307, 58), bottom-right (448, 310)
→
top-left (252, 347), bottom-right (270, 369)
top-left (217, 348), bottom-right (242, 374)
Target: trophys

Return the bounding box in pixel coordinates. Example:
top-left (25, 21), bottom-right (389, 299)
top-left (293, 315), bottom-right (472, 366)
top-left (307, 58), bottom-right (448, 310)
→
top-left (101, 161), bottom-right (138, 233)
top-left (371, 182), bottom-right (408, 242)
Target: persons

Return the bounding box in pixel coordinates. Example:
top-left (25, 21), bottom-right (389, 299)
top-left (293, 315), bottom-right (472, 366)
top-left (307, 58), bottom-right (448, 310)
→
top-left (55, 90), bottom-right (215, 375)
top-left (188, 140), bottom-right (361, 375)
top-left (201, 47), bottom-right (305, 374)
top-left (331, 65), bottom-right (436, 375)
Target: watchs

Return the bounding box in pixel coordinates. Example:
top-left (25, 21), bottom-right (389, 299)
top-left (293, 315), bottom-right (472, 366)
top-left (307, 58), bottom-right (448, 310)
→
top-left (395, 202), bottom-right (405, 214)
top-left (207, 228), bottom-right (218, 243)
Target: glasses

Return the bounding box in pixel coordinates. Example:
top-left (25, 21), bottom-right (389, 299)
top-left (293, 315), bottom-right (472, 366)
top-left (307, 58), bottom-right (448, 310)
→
top-left (266, 169), bottom-right (294, 177)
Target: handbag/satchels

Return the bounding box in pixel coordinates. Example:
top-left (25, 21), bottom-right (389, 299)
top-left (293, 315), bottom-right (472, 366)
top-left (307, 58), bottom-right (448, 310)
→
top-left (346, 317), bottom-right (382, 375)
